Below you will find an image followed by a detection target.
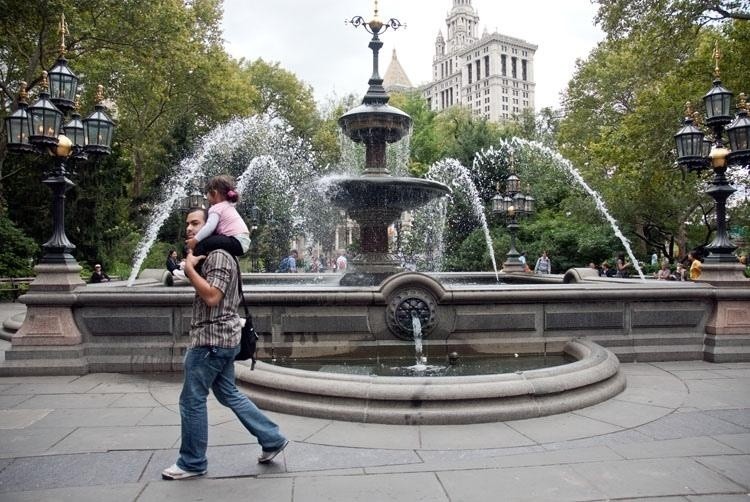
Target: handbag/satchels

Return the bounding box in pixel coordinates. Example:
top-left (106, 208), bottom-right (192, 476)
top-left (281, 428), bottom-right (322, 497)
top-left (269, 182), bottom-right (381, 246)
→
top-left (235, 317), bottom-right (260, 371)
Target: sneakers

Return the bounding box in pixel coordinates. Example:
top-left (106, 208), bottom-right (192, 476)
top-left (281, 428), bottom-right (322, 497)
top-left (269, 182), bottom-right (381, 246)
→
top-left (257, 437), bottom-right (290, 464)
top-left (161, 463), bottom-right (208, 480)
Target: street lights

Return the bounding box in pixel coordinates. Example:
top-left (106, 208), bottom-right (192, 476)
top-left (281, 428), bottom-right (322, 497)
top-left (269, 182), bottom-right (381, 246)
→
top-left (673, 40), bottom-right (750, 263)
top-left (491, 151), bottom-right (535, 262)
top-left (5, 13), bottom-right (115, 265)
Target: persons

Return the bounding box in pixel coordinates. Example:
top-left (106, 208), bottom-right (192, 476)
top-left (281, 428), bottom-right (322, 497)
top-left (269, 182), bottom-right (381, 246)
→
top-left (286, 249), bottom-right (298, 272)
top-left (670, 262), bottom-right (687, 281)
top-left (599, 261), bottom-right (614, 277)
top-left (309, 254), bottom-right (320, 273)
top-left (519, 250), bottom-right (532, 272)
top-left (688, 250), bottom-right (703, 281)
top-left (534, 250), bottom-right (551, 274)
top-left (182, 175), bottom-right (251, 277)
top-left (304, 252), bottom-right (313, 273)
top-left (337, 252), bottom-right (348, 272)
top-left (330, 255), bottom-right (337, 272)
top-left (89, 263), bottom-right (110, 283)
top-left (166, 249), bottom-right (180, 273)
top-left (652, 260), bottom-right (671, 280)
top-left (615, 251), bottom-right (632, 278)
top-left (161, 206), bottom-right (291, 481)
